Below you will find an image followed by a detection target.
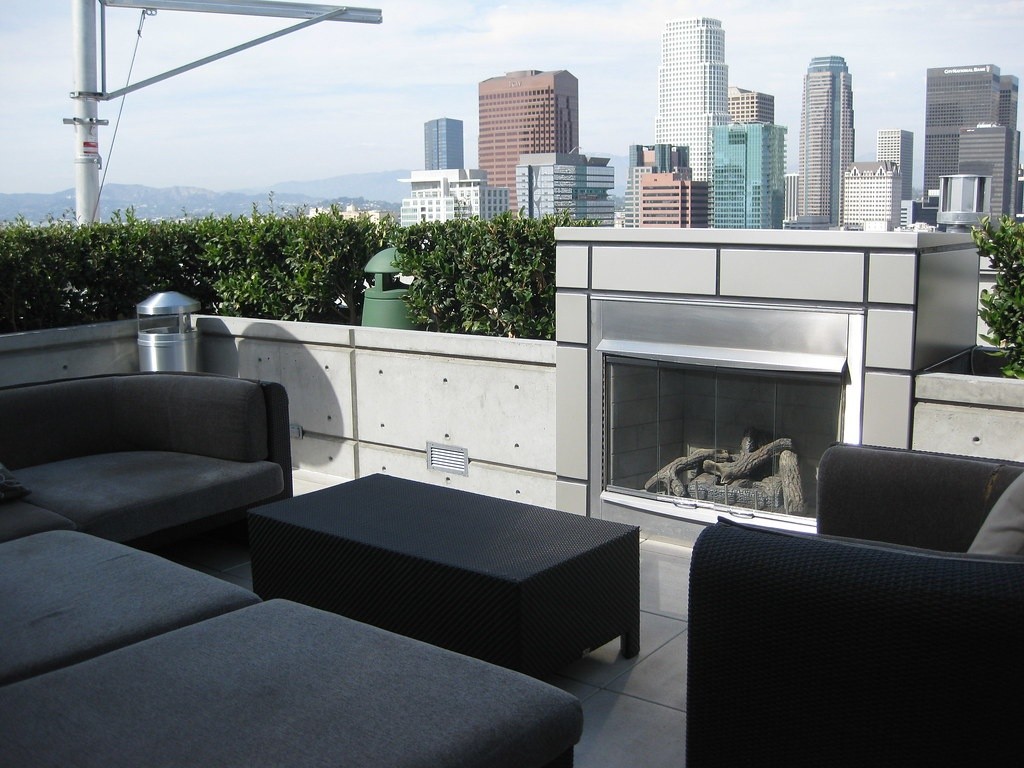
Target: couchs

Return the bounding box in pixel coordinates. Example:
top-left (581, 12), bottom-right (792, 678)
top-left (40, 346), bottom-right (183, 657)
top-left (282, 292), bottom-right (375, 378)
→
top-left (0, 371), bottom-right (583, 768)
top-left (686, 443), bottom-right (1024, 768)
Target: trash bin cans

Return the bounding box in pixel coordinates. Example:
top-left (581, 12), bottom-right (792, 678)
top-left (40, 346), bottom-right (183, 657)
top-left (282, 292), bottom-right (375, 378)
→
top-left (361, 246), bottom-right (427, 331)
top-left (134, 293), bottom-right (204, 372)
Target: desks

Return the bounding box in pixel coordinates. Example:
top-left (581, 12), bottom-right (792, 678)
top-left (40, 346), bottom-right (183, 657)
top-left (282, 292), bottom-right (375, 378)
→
top-left (247, 474), bottom-right (639, 679)
